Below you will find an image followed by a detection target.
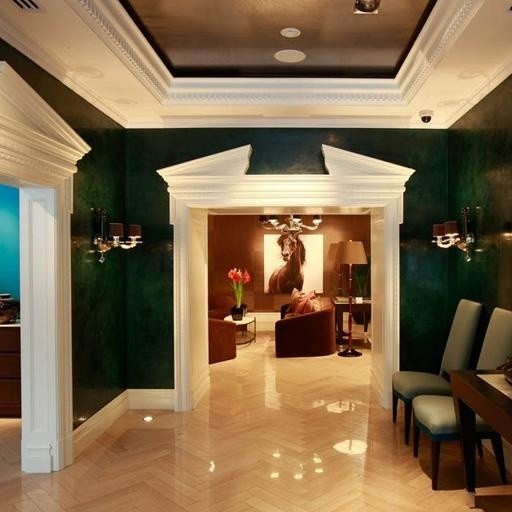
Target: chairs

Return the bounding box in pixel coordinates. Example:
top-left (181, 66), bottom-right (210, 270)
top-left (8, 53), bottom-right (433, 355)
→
top-left (391, 298), bottom-right (484, 444)
top-left (411, 304), bottom-right (512, 490)
top-left (208, 309), bottom-right (236, 364)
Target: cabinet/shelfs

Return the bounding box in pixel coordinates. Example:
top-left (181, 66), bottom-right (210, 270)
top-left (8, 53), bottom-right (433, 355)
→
top-left (1, 323), bottom-right (22, 418)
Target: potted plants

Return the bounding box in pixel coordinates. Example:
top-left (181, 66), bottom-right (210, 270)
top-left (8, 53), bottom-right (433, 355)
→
top-left (350, 256), bottom-right (371, 325)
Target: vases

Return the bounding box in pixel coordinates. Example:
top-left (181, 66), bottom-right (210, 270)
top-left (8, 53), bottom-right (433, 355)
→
top-left (231, 304), bottom-right (247, 320)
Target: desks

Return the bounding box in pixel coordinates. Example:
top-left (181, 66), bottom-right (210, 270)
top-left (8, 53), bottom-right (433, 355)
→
top-left (441, 368), bottom-right (512, 512)
top-left (331, 296), bottom-right (372, 343)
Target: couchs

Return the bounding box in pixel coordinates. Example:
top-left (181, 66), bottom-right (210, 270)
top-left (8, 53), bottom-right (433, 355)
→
top-left (274, 295), bottom-right (335, 357)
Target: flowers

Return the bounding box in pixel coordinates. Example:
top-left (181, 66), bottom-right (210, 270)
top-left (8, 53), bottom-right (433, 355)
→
top-left (227, 268), bottom-right (251, 307)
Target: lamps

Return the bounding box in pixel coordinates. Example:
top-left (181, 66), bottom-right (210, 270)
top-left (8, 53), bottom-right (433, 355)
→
top-left (336, 239), bottom-right (367, 358)
top-left (429, 207), bottom-right (476, 263)
top-left (328, 243), bottom-right (348, 337)
top-left (91, 206), bottom-right (143, 263)
top-left (256, 215), bottom-right (322, 232)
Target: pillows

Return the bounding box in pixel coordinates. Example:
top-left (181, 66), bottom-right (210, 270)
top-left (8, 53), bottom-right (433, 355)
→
top-left (286, 287), bottom-right (321, 317)
